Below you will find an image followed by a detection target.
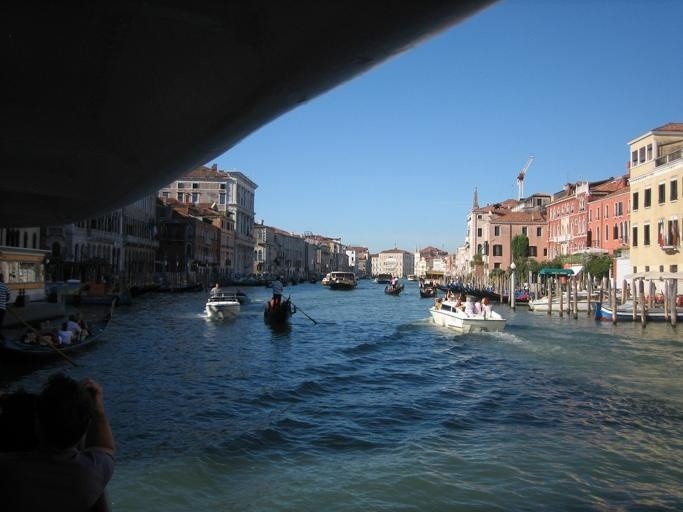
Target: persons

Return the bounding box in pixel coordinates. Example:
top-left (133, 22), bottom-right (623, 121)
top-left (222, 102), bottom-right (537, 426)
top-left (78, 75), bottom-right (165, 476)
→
top-left (0, 371), bottom-right (117, 512)
top-left (391, 276), bottom-right (396, 288)
top-left (468, 281), bottom-right (471, 288)
top-left (268, 277), bottom-right (284, 306)
top-left (433, 278), bottom-right (436, 286)
top-left (0, 273), bottom-right (12, 343)
top-left (418, 275), bottom-right (425, 288)
top-left (628, 283), bottom-right (630, 297)
top-left (207, 282), bottom-right (225, 302)
top-left (453, 281), bottom-right (458, 287)
top-left (24, 312), bottom-right (89, 346)
top-left (434, 288), bottom-right (490, 314)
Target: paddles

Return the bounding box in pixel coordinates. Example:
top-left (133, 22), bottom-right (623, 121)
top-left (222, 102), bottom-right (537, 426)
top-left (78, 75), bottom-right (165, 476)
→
top-left (6, 305), bottom-right (87, 367)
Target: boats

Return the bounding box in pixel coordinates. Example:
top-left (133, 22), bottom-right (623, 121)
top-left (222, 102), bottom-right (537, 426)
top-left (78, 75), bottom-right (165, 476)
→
top-left (529, 290), bottom-right (622, 312)
top-left (384, 285), bottom-right (404, 295)
top-left (420, 287), bottom-right (437, 299)
top-left (408, 274), bottom-right (417, 281)
top-left (204, 296), bottom-right (241, 323)
top-left (320, 271), bottom-right (357, 289)
top-left (0, 298), bottom-right (118, 357)
top-left (377, 273), bottom-right (392, 284)
top-left (263, 296), bottom-right (294, 323)
top-left (601, 300), bottom-right (683, 322)
top-left (428, 298), bottom-right (508, 336)
